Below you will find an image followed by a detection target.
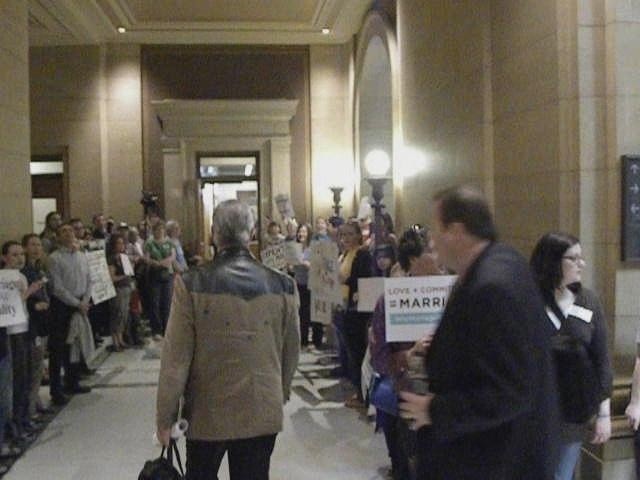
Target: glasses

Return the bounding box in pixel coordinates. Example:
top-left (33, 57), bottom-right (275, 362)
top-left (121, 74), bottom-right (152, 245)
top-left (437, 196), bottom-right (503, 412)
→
top-left (560, 254), bottom-right (585, 262)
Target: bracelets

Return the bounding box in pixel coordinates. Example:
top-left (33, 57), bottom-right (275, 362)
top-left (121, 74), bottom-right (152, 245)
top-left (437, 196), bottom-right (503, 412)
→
top-left (598, 414), bottom-right (611, 417)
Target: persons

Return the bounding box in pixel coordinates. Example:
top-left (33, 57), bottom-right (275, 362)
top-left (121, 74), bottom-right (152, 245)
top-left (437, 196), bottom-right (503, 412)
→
top-left (1, 211), bottom-right (188, 475)
top-left (293, 222), bottom-right (312, 345)
top-left (157, 199), bottom-right (302, 480)
top-left (624, 328), bottom-right (640, 480)
top-left (310, 213), bottom-right (395, 419)
top-left (267, 221), bottom-right (285, 247)
top-left (397, 186), bottom-right (563, 479)
top-left (367, 224), bottom-right (446, 479)
top-left (285, 218), bottom-right (299, 278)
top-left (530, 231), bottom-right (614, 480)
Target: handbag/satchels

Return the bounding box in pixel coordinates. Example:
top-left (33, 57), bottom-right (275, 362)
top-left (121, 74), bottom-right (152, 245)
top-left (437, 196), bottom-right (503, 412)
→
top-left (138, 456), bottom-right (186, 480)
top-left (366, 361), bottom-right (403, 417)
